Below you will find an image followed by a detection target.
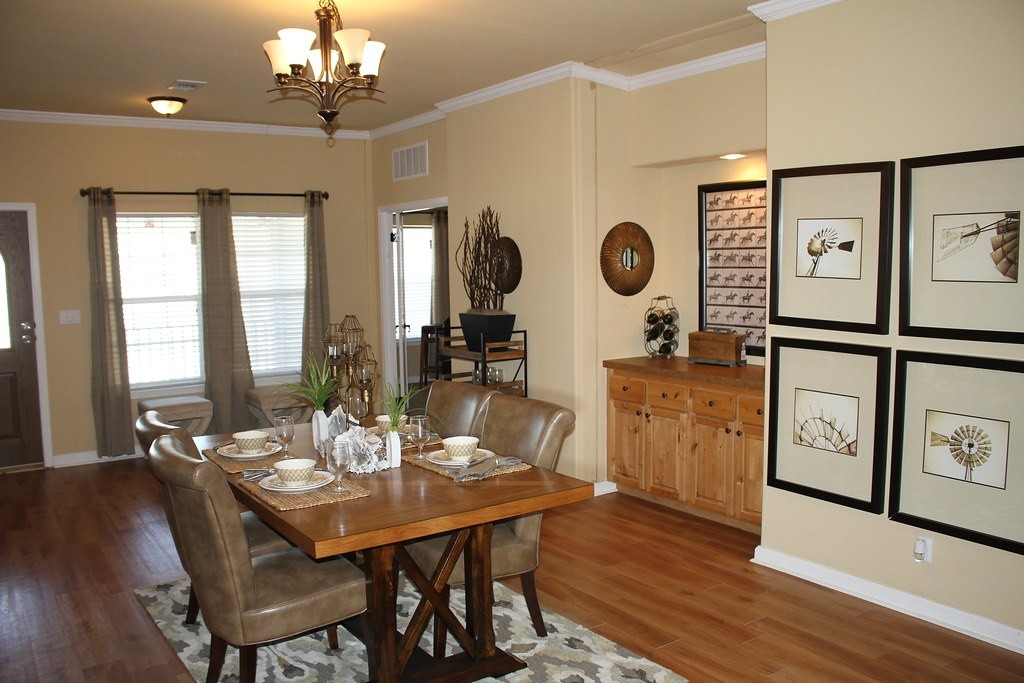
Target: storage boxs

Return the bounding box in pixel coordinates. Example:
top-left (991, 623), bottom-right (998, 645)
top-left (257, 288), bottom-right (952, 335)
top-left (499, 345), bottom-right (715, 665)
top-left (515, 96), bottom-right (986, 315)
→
top-left (689, 331), bottom-right (747, 367)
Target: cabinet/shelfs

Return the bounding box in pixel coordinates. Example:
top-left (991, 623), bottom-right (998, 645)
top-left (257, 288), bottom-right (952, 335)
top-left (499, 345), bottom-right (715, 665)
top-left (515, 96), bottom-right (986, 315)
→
top-left (434, 326), bottom-right (529, 399)
top-left (602, 355), bottom-right (766, 537)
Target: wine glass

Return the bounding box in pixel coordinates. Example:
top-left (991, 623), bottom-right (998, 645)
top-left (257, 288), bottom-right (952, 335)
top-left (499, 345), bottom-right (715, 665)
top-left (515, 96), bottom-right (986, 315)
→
top-left (326, 442), bottom-right (351, 493)
top-left (409, 415), bottom-right (431, 460)
top-left (274, 415), bottom-right (296, 460)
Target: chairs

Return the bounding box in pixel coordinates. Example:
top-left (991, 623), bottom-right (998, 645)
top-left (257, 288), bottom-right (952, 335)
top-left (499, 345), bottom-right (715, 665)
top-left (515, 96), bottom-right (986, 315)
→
top-left (424, 381), bottom-right (502, 449)
top-left (135, 410), bottom-right (294, 626)
top-left (399, 393), bottom-right (577, 654)
top-left (149, 432), bottom-right (377, 683)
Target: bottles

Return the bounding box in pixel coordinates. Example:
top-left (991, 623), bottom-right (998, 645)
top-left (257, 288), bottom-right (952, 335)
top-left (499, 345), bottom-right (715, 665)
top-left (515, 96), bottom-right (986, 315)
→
top-left (646, 309), bottom-right (678, 355)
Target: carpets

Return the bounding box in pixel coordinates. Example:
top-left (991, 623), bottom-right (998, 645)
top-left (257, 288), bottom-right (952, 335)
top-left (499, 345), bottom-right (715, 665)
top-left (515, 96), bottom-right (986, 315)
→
top-left (132, 575), bottom-right (690, 683)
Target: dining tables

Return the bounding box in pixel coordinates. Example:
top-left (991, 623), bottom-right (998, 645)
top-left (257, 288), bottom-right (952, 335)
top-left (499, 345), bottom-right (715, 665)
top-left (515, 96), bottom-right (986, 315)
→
top-left (191, 413), bottom-right (596, 683)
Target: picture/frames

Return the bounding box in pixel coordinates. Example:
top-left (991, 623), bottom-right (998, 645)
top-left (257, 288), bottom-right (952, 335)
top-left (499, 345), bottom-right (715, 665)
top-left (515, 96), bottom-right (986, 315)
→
top-left (888, 349), bottom-right (1024, 555)
top-left (768, 160), bottom-right (896, 335)
top-left (898, 145), bottom-right (1024, 345)
top-left (766, 336), bottom-right (892, 516)
top-left (697, 180), bottom-right (767, 356)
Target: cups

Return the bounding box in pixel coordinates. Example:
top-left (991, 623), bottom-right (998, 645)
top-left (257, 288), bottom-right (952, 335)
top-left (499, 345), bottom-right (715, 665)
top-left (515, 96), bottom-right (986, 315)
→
top-left (496, 369), bottom-right (504, 383)
top-left (471, 369), bottom-right (482, 385)
top-left (486, 367), bottom-right (496, 384)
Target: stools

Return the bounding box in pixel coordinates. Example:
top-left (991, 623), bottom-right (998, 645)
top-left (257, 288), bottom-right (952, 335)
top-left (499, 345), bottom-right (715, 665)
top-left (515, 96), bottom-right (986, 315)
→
top-left (137, 395), bottom-right (213, 436)
top-left (246, 383), bottom-right (316, 430)
top-left (419, 325), bottom-right (445, 384)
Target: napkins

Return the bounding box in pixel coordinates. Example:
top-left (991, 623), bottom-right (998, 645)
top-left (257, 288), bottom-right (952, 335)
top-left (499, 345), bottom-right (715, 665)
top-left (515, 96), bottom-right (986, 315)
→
top-left (453, 455), bottom-right (522, 484)
top-left (380, 431), bottom-right (438, 444)
top-left (213, 437), bottom-right (278, 450)
top-left (242, 465), bottom-right (329, 482)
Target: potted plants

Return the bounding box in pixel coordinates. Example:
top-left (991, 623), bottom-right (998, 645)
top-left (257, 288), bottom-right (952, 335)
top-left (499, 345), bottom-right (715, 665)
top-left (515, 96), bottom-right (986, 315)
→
top-left (271, 348), bottom-right (348, 450)
top-left (454, 204), bottom-right (518, 353)
top-left (365, 380), bottom-right (432, 469)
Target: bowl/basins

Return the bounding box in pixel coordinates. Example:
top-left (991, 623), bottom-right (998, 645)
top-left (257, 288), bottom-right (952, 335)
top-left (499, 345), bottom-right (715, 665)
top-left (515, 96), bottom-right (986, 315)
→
top-left (375, 415), bottom-right (408, 433)
top-left (232, 431), bottom-right (269, 454)
top-left (274, 458), bottom-right (316, 487)
top-left (442, 436), bottom-right (479, 460)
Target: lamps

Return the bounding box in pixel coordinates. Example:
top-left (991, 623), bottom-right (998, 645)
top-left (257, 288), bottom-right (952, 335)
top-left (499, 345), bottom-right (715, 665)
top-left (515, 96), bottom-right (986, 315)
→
top-left (321, 314), bottom-right (379, 419)
top-left (263, 0), bottom-right (387, 135)
top-left (146, 96), bottom-right (188, 119)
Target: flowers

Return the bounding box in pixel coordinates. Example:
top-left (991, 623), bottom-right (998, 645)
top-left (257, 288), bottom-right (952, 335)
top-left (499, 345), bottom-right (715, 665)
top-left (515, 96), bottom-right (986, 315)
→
top-left (330, 425), bottom-right (383, 474)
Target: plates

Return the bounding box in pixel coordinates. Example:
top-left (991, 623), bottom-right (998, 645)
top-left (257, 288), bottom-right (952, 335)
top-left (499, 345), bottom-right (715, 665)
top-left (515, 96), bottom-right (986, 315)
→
top-left (366, 425), bottom-right (410, 436)
top-left (259, 471), bottom-right (335, 495)
top-left (216, 442), bottom-right (283, 461)
top-left (426, 449), bottom-right (495, 469)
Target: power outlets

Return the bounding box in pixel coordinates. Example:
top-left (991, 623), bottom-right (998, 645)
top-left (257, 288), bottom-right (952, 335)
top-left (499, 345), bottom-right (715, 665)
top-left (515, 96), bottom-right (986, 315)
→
top-left (917, 535), bottom-right (934, 564)
